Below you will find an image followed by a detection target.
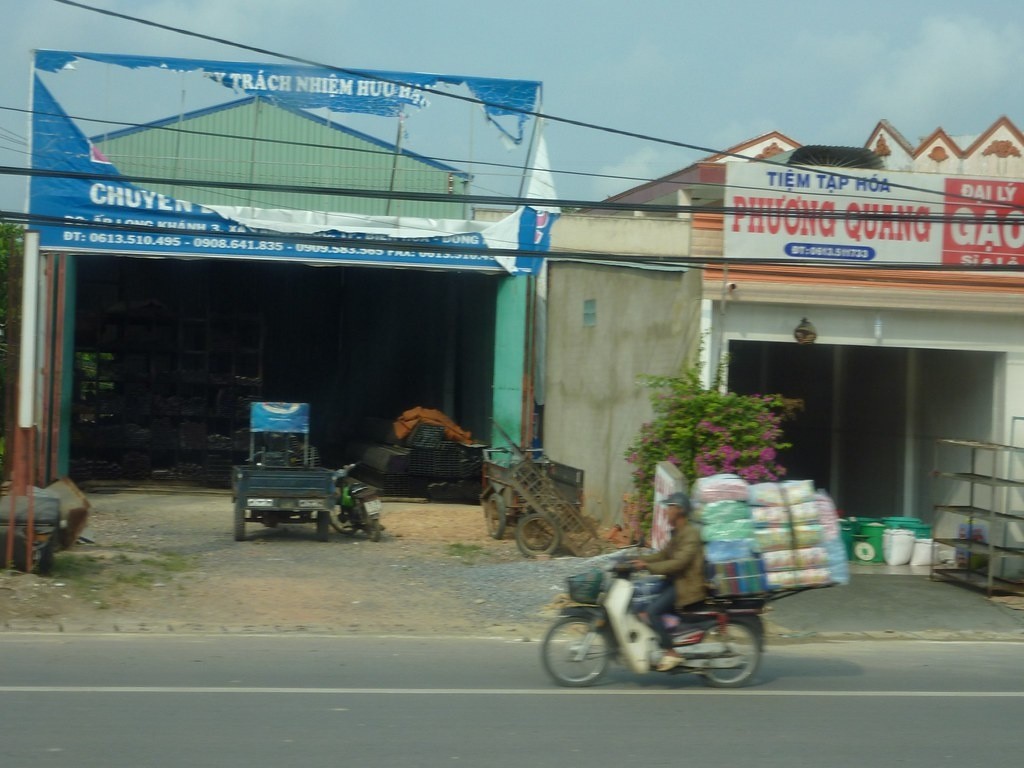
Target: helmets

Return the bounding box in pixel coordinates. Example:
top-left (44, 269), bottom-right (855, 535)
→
top-left (660, 492), bottom-right (690, 508)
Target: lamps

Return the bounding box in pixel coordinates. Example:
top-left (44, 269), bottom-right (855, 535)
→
top-left (795, 318), bottom-right (818, 346)
top-left (873, 321), bottom-right (885, 341)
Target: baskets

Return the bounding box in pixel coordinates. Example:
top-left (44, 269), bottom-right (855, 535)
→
top-left (565, 572), bottom-right (603, 604)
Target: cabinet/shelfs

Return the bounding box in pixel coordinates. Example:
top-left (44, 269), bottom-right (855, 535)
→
top-left (928, 439), bottom-right (1024, 602)
top-left (70, 306), bottom-right (264, 488)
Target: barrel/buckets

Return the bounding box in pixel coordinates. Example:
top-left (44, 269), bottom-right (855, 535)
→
top-left (836, 514), bottom-right (932, 566)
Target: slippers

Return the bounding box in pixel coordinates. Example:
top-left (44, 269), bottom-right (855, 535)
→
top-left (656, 655), bottom-right (686, 671)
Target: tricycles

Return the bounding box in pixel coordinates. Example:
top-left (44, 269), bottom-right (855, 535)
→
top-left (482, 448), bottom-right (586, 538)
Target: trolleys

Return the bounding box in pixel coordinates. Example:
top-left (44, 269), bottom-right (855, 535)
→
top-left (484, 416), bottom-right (604, 559)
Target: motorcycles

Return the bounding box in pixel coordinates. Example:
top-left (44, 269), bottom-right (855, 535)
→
top-left (331, 461), bottom-right (385, 543)
top-left (541, 565), bottom-right (768, 688)
top-left (228, 433), bottom-right (335, 542)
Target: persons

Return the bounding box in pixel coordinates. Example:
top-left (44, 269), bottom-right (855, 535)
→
top-left (630, 492), bottom-right (705, 671)
